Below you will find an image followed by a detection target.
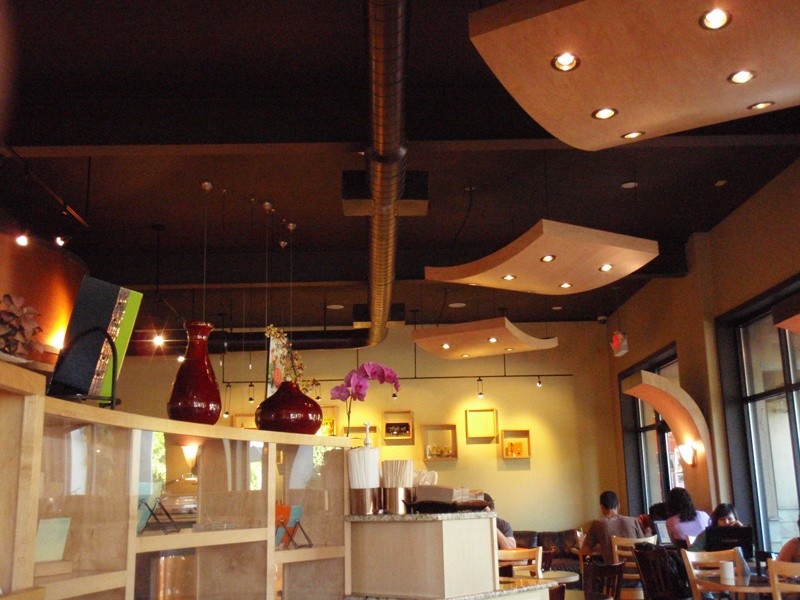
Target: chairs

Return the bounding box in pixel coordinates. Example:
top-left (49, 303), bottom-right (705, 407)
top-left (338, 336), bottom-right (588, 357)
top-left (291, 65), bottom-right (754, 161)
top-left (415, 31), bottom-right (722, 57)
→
top-left (496, 529), bottom-right (800, 600)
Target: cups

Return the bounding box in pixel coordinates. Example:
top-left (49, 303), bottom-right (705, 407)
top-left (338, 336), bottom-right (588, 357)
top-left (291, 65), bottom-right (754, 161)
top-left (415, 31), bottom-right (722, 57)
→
top-left (719, 560), bottom-right (735, 586)
top-left (349, 487), bottom-right (382, 515)
top-left (382, 488), bottom-right (414, 515)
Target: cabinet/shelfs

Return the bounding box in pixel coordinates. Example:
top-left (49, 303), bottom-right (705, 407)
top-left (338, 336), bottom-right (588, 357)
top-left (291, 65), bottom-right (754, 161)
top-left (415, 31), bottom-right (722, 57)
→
top-left (0, 361), bottom-right (355, 600)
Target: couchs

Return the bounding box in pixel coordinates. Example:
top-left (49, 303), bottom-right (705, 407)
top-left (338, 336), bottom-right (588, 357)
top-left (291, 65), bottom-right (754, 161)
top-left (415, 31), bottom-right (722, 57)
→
top-left (513, 531), bottom-right (583, 588)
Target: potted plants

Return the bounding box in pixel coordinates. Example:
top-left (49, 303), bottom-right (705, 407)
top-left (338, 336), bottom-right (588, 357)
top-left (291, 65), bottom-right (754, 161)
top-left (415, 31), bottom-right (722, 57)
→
top-left (0, 294), bottom-right (44, 365)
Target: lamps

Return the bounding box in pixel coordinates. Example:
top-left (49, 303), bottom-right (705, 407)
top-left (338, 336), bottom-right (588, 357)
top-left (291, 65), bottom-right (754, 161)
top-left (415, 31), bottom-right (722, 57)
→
top-left (55, 232), bottom-right (72, 247)
top-left (149, 183), bottom-right (630, 420)
top-left (14, 229), bottom-right (30, 247)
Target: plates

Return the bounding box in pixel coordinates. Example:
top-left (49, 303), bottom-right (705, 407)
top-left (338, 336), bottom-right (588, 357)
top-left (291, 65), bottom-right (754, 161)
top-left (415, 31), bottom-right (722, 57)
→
top-left (401, 498), bottom-right (494, 513)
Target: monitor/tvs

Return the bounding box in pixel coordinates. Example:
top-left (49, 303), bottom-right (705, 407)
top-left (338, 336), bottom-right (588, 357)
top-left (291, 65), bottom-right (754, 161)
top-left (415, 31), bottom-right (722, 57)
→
top-left (705, 526), bottom-right (753, 559)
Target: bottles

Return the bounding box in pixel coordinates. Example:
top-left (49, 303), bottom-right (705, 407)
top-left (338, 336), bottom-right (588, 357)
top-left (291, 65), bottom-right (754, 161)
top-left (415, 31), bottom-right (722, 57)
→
top-left (167, 321), bottom-right (221, 423)
top-left (254, 380), bottom-right (323, 435)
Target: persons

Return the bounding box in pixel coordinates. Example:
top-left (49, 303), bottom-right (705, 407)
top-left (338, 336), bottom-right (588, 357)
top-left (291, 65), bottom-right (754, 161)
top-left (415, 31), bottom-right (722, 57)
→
top-left (766, 518), bottom-right (800, 600)
top-left (643, 487), bottom-right (755, 600)
top-left (484, 493), bottom-right (516, 584)
top-left (580, 491), bottom-right (645, 589)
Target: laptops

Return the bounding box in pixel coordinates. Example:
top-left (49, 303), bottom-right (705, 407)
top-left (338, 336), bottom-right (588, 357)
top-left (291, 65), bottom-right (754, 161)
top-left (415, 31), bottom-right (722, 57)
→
top-left (653, 520), bottom-right (677, 549)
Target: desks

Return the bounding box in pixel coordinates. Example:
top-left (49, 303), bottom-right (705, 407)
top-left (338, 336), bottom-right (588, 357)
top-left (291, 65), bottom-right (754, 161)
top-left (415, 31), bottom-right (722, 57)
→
top-left (542, 569), bottom-right (580, 583)
top-left (698, 574), bottom-right (795, 600)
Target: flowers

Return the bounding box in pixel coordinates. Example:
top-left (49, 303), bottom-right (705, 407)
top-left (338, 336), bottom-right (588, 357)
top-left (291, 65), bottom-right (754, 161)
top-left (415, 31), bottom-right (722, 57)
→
top-left (329, 363), bottom-right (400, 438)
top-left (264, 325), bottom-right (321, 396)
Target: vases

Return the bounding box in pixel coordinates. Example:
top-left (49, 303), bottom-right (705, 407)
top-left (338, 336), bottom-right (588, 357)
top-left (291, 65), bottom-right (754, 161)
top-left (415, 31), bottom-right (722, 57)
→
top-left (167, 320), bottom-right (222, 426)
top-left (254, 381), bottom-right (323, 434)
